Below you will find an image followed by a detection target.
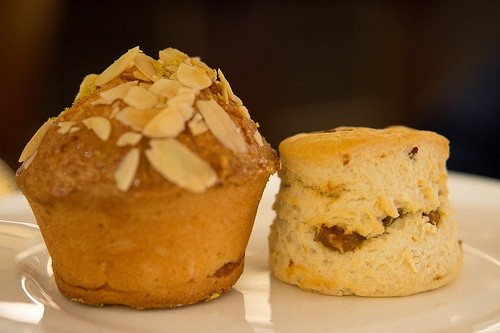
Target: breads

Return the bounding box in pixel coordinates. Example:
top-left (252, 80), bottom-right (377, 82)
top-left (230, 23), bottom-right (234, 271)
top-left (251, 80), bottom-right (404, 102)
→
top-left (269, 126), bottom-right (464, 297)
top-left (15, 47), bottom-right (279, 309)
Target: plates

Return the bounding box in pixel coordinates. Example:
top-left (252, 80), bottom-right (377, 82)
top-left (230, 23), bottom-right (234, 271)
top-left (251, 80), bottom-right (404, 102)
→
top-left (0, 170), bottom-right (500, 333)
